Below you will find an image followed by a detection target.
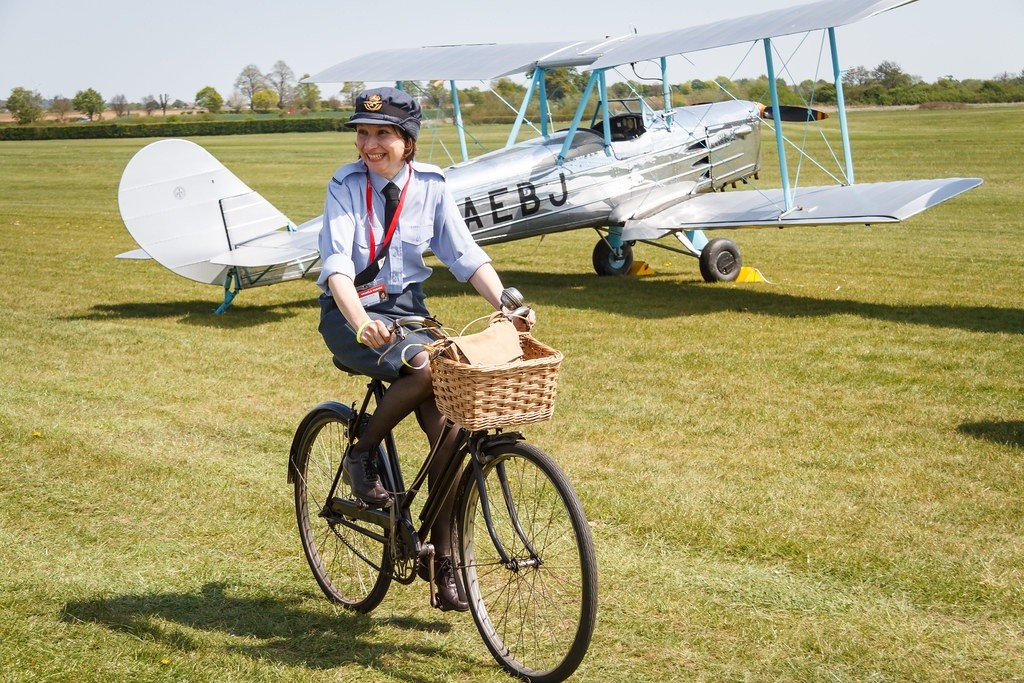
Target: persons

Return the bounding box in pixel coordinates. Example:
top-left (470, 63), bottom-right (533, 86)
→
top-left (315, 87), bottom-right (536, 612)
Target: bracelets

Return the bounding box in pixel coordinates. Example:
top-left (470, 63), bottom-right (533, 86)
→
top-left (356, 320), bottom-right (375, 343)
top-left (499, 303), bottom-right (505, 311)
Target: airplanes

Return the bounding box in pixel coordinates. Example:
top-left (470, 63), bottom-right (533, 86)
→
top-left (116, 0), bottom-right (985, 314)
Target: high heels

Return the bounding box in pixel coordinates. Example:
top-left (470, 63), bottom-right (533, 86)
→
top-left (342, 444), bottom-right (389, 504)
top-left (419, 551), bottom-right (469, 613)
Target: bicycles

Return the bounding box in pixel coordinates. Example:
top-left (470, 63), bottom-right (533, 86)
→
top-left (285, 286), bottom-right (599, 683)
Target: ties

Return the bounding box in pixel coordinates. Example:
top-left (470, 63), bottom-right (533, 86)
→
top-left (352, 183), bottom-right (400, 287)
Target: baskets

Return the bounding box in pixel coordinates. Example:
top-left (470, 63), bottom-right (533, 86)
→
top-left (428, 329), bottom-right (565, 431)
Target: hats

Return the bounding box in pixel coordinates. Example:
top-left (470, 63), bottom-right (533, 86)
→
top-left (344, 86), bottom-right (423, 143)
